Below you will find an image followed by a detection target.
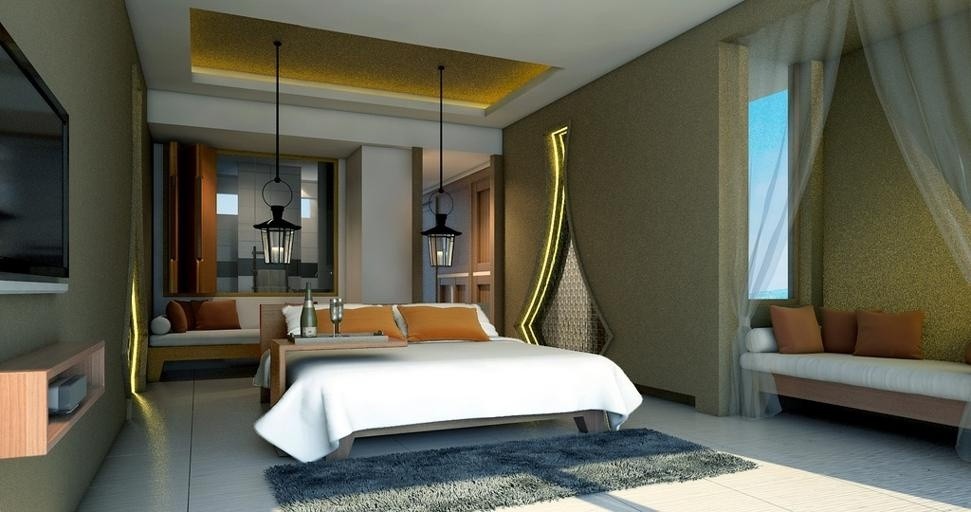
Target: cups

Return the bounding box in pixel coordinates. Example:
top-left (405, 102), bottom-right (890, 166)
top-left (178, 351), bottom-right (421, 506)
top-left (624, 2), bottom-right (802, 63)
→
top-left (328, 297), bottom-right (350, 338)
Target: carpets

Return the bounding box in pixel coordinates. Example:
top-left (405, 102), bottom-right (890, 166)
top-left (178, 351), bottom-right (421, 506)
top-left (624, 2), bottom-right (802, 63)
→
top-left (261, 427), bottom-right (756, 512)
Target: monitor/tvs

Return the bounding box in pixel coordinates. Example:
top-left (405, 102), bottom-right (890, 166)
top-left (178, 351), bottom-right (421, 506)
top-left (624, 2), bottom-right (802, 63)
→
top-left (1, 23), bottom-right (71, 294)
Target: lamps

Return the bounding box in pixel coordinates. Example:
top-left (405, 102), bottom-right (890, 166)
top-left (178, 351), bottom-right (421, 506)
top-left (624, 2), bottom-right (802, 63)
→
top-left (422, 67), bottom-right (463, 269)
top-left (253, 36), bottom-right (302, 264)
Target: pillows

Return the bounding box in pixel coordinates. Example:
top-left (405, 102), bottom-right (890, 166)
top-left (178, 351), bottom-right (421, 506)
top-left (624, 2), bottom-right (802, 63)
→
top-left (392, 302), bottom-right (500, 339)
top-left (856, 311), bottom-right (925, 359)
top-left (400, 305), bottom-right (490, 344)
top-left (192, 298), bottom-right (241, 329)
top-left (282, 302), bottom-right (398, 337)
top-left (317, 305), bottom-right (405, 340)
top-left (153, 314), bottom-right (173, 337)
top-left (770, 303), bottom-right (824, 353)
top-left (165, 300), bottom-right (188, 333)
top-left (822, 307), bottom-right (885, 353)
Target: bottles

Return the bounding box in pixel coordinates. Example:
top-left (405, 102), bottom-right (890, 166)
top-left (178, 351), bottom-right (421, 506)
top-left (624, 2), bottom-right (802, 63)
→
top-left (300, 279), bottom-right (318, 338)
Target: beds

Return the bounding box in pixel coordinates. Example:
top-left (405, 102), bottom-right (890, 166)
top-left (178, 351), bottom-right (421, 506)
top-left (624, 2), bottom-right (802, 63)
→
top-left (251, 301), bottom-right (644, 463)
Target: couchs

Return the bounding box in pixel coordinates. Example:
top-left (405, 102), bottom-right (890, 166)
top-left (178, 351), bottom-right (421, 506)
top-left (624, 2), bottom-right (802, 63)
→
top-left (146, 296), bottom-right (338, 383)
top-left (739, 325), bottom-right (971, 428)
